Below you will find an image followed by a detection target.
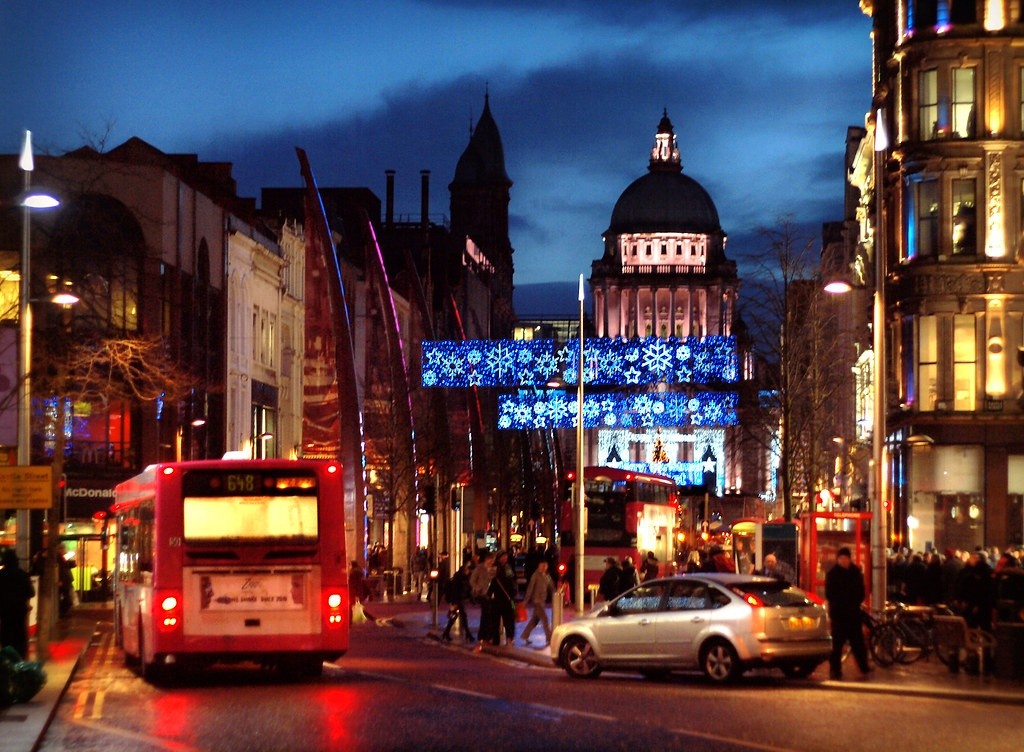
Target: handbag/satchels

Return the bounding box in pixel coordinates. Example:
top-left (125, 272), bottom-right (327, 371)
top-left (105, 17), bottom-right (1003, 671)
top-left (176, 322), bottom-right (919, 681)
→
top-left (514, 602), bottom-right (529, 622)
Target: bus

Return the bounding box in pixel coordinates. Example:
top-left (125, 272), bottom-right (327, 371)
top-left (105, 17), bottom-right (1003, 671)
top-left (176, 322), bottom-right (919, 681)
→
top-left (557, 465), bottom-right (679, 605)
top-left (110, 451), bottom-right (349, 681)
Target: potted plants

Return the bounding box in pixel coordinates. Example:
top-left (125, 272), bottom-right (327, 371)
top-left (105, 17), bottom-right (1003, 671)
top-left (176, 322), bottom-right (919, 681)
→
top-left (4, 646), bottom-right (47, 703)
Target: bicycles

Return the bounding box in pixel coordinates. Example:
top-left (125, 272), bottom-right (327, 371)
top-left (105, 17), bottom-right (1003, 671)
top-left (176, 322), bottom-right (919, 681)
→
top-left (841, 601), bottom-right (995, 678)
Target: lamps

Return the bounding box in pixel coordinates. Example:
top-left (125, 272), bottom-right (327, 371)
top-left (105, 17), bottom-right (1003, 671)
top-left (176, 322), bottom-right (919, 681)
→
top-left (986, 399), bottom-right (1003, 413)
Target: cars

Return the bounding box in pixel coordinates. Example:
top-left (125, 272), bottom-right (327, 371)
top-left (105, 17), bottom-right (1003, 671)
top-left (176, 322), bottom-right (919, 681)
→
top-left (550, 572), bottom-right (833, 686)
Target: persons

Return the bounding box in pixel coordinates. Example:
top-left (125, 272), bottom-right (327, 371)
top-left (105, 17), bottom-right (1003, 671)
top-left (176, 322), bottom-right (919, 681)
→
top-left (0, 549), bottom-right (35, 660)
top-left (599, 546), bottom-right (735, 601)
top-left (411, 541), bottom-right (575, 648)
top-left (31, 544), bottom-right (77, 618)
top-left (759, 554), bottom-right (785, 580)
top-left (825, 547), bottom-right (871, 678)
top-left (886, 543), bottom-right (1024, 614)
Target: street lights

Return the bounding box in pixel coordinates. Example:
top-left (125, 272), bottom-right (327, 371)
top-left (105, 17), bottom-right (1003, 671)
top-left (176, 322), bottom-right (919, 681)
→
top-left (824, 108), bottom-right (889, 619)
top-left (15, 129), bottom-right (79, 574)
top-left (547, 273), bottom-right (585, 614)
